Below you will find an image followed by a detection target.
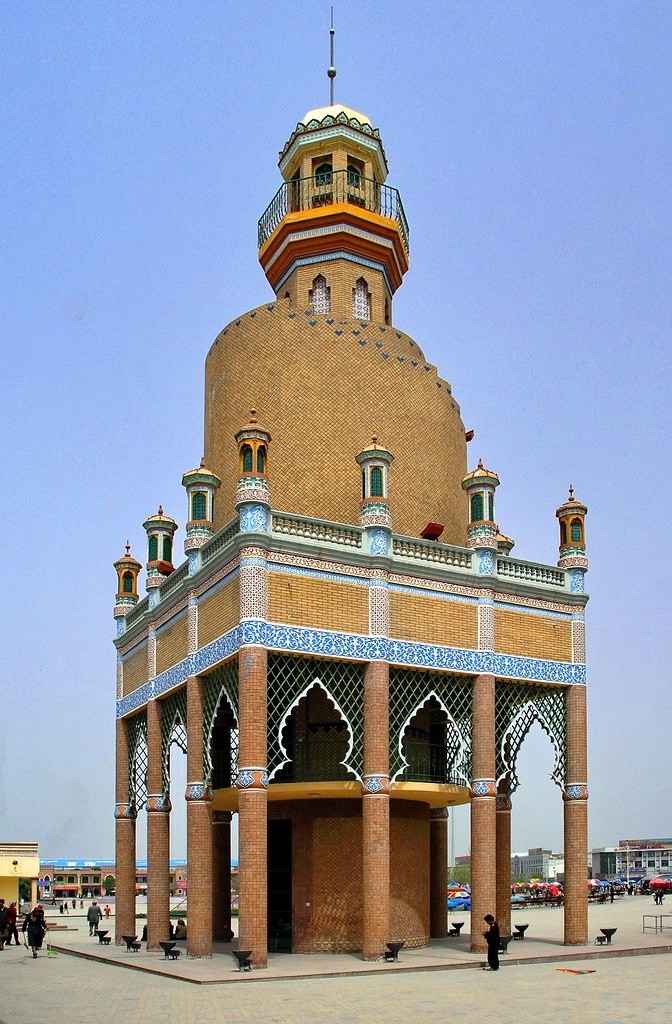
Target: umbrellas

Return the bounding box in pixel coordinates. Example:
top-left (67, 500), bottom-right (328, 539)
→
top-left (651, 879), bottom-right (672, 888)
top-left (588, 878), bottom-right (636, 888)
top-left (511, 880), bottom-right (563, 888)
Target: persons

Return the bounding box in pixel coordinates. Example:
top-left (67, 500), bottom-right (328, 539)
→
top-left (87, 901), bottom-right (102, 936)
top-left (141, 918), bottom-right (187, 941)
top-left (653, 890), bottom-right (663, 906)
top-left (0, 898), bottom-right (48, 958)
top-left (482, 915), bottom-right (501, 971)
top-left (52, 897), bottom-right (84, 914)
top-left (611, 887), bottom-right (614, 903)
top-left (104, 905), bottom-right (111, 918)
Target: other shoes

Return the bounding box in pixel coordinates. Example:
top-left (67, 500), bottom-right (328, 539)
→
top-left (487, 968), bottom-right (497, 971)
top-left (94, 935), bottom-right (97, 936)
top-left (6, 942), bottom-right (14, 946)
top-left (33, 954), bottom-right (37, 959)
top-left (0, 946), bottom-right (4, 950)
top-left (90, 933), bottom-right (93, 936)
top-left (16, 942), bottom-right (21, 945)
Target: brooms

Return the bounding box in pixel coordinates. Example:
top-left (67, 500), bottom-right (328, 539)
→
top-left (22, 931), bottom-right (29, 951)
